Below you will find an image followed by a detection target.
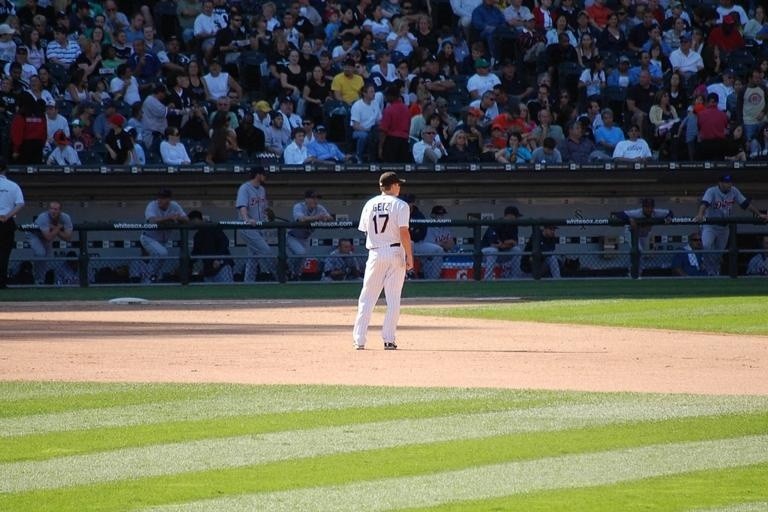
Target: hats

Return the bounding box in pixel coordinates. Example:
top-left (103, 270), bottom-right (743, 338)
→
top-left (404, 193), bottom-right (414, 202)
top-left (254, 166), bottom-right (270, 174)
top-left (473, 58), bottom-right (490, 69)
top-left (504, 206), bottom-right (523, 217)
top-left (678, 33), bottom-right (693, 41)
top-left (305, 189), bottom-right (321, 198)
top-left (255, 101), bottom-right (272, 113)
top-left (0, 24), bottom-right (16, 35)
top-left (380, 172), bottom-right (406, 187)
top-left (432, 206), bottom-right (446, 214)
top-left (45, 98), bottom-right (137, 144)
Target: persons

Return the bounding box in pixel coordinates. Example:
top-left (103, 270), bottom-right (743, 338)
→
top-left (140, 189), bottom-right (190, 281)
top-left (1, 1), bottom-right (767, 168)
top-left (29, 199), bottom-right (74, 284)
top-left (287, 190), bottom-right (335, 279)
top-left (691, 172), bottom-right (767, 275)
top-left (186, 210), bottom-right (234, 283)
top-left (611, 197), bottom-right (673, 276)
top-left (324, 238), bottom-right (362, 279)
top-left (0, 164), bottom-right (25, 287)
top-left (402, 192), bottom-right (563, 279)
top-left (237, 167), bottom-right (283, 285)
top-left (351, 172), bottom-right (414, 349)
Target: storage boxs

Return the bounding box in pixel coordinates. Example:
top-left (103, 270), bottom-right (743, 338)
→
top-left (479, 262), bottom-right (505, 278)
top-left (438, 261), bottom-right (472, 280)
top-left (300, 258), bottom-right (320, 272)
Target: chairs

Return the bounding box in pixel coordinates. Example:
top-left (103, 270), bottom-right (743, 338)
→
top-left (0, 2), bottom-right (764, 163)
